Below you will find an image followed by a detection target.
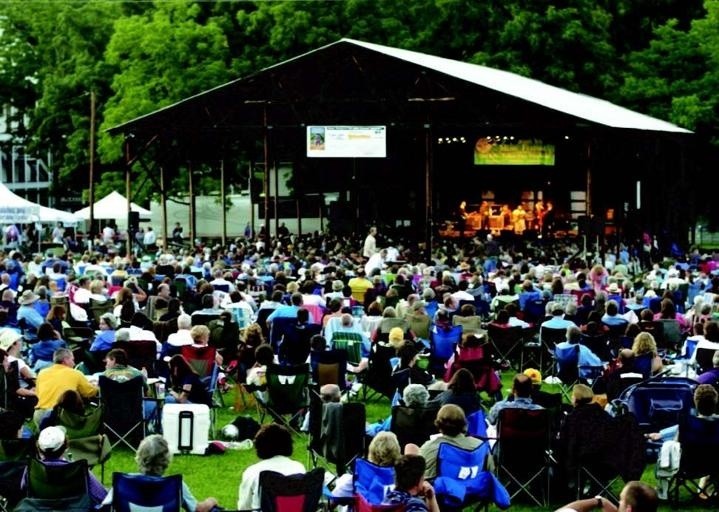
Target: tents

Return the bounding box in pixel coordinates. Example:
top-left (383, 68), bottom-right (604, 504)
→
top-left (72, 189), bottom-right (156, 235)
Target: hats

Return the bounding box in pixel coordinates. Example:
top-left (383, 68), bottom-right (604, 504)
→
top-left (523, 368), bottom-right (541, 385)
top-left (18, 289), bottom-right (40, 305)
top-left (0, 328), bottom-right (24, 352)
top-left (38, 425), bottom-right (67, 453)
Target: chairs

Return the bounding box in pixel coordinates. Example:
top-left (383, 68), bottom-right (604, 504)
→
top-left (0, 234), bottom-right (719, 511)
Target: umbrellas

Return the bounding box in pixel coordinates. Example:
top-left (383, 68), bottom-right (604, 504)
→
top-left (1, 182), bottom-right (81, 226)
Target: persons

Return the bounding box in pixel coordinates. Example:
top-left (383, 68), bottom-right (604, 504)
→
top-left (0, 201), bottom-right (718, 512)
top-left (315, 132), bottom-right (320, 150)
